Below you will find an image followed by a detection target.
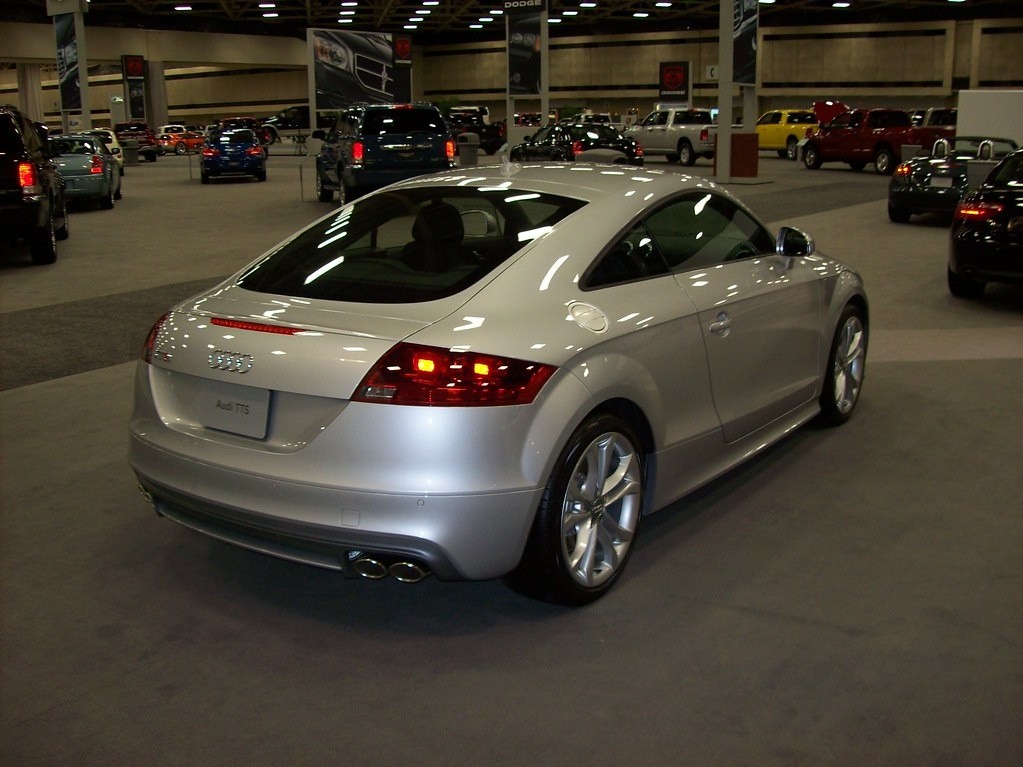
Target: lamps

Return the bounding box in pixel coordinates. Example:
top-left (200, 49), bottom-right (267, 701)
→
top-left (174, 4), bottom-right (192, 10)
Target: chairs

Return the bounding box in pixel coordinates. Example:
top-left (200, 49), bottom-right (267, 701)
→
top-left (931, 138), bottom-right (951, 158)
top-left (399, 202), bottom-right (480, 273)
top-left (976, 139), bottom-right (995, 161)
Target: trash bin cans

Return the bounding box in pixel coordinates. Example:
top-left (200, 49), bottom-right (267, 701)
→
top-left (457, 133), bottom-right (480, 165)
top-left (122, 139), bottom-right (141, 164)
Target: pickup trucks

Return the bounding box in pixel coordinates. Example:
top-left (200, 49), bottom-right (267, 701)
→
top-left (802, 101), bottom-right (956, 177)
top-left (621, 109), bottom-right (742, 167)
top-left (262, 104), bottom-right (343, 143)
top-left (565, 113), bottom-right (627, 133)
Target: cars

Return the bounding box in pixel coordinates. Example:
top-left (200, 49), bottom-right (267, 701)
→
top-left (46, 133), bottom-right (121, 208)
top-left (199, 128), bottom-right (266, 185)
top-left (922, 107), bottom-right (958, 130)
top-left (80, 129), bottom-right (125, 176)
top-left (756, 108), bottom-right (819, 160)
top-left (0, 104), bottom-right (68, 266)
top-left (126, 157), bottom-right (874, 612)
top-left (508, 121), bottom-right (644, 166)
top-left (448, 105), bottom-right (560, 155)
top-left (558, 119), bottom-right (571, 125)
top-left (947, 148), bottom-right (1023, 298)
top-left (909, 109), bottom-right (927, 127)
top-left (205, 125), bottom-right (216, 136)
top-left (887, 136), bottom-right (1020, 221)
top-left (157, 125), bottom-right (205, 154)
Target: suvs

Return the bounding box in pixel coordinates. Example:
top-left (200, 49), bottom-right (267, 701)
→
top-left (113, 121), bottom-right (156, 162)
top-left (208, 117), bottom-right (271, 160)
top-left (311, 101), bottom-right (455, 205)
